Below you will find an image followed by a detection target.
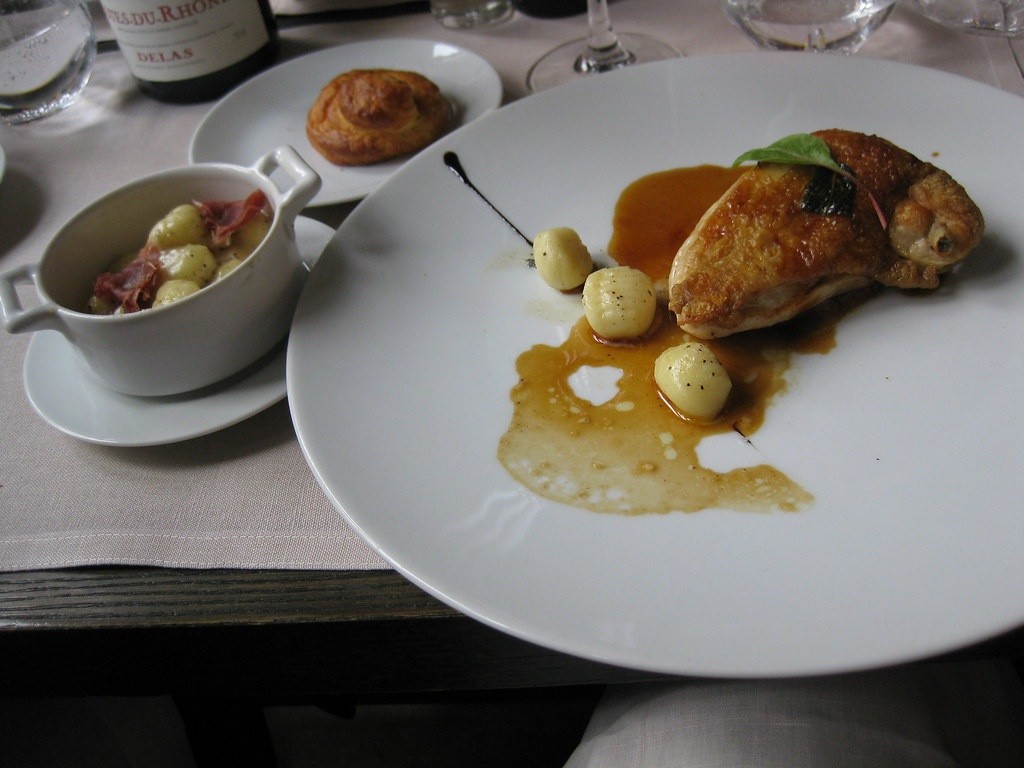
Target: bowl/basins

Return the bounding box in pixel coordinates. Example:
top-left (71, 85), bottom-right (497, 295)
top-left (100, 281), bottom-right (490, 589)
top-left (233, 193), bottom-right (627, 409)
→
top-left (0, 148), bottom-right (322, 399)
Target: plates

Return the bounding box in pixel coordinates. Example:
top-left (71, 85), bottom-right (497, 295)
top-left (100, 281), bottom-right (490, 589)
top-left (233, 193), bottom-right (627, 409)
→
top-left (285, 56), bottom-right (1024, 679)
top-left (22, 213), bottom-right (337, 446)
top-left (187, 37), bottom-right (501, 208)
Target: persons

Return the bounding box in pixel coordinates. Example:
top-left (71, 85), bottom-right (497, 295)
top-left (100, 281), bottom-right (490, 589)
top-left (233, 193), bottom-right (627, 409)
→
top-left (563, 689), bottom-right (962, 767)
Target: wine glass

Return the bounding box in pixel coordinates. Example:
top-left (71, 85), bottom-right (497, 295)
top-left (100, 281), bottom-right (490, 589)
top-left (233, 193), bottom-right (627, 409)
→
top-left (525, 0), bottom-right (682, 97)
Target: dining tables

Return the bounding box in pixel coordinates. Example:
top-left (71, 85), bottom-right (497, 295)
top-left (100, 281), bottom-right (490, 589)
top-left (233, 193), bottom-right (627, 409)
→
top-left (2, 1), bottom-right (1023, 768)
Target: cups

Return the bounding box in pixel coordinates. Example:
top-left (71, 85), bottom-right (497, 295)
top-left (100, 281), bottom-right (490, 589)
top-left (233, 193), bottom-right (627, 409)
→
top-left (0, 0), bottom-right (96, 127)
top-left (430, 0), bottom-right (515, 30)
top-left (720, 0), bottom-right (899, 56)
top-left (906, 0), bottom-right (1024, 39)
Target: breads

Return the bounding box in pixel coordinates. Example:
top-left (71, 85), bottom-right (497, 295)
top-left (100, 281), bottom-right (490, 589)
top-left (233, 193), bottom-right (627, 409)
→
top-left (307, 68), bottom-right (451, 166)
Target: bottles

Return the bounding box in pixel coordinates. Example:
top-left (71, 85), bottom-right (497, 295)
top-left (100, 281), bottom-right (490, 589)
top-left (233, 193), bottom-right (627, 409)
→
top-left (98, 0), bottom-right (281, 107)
